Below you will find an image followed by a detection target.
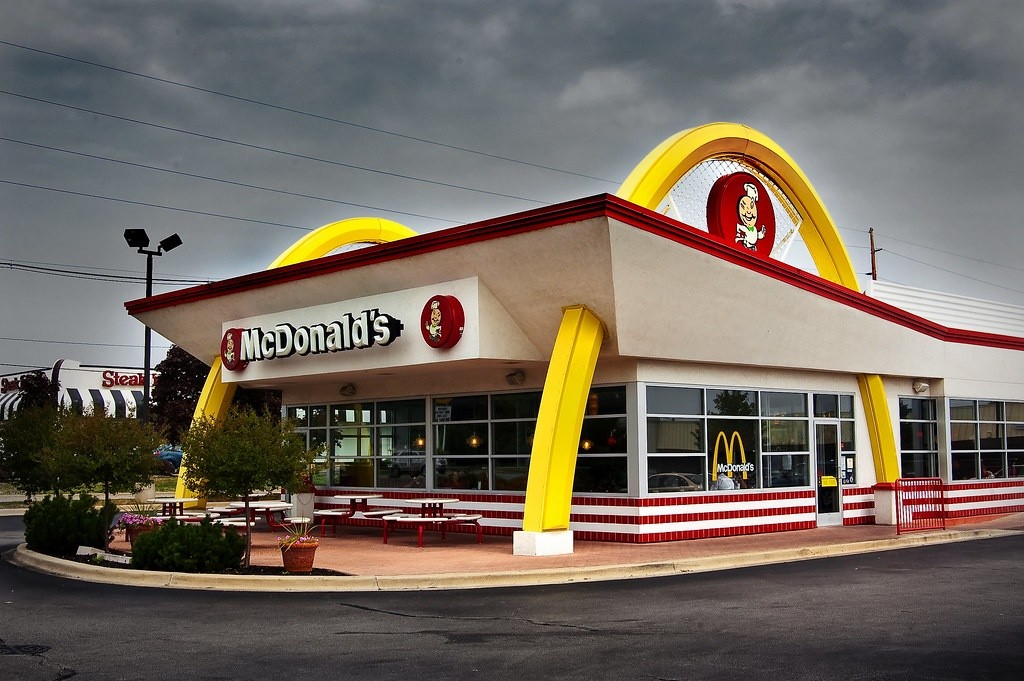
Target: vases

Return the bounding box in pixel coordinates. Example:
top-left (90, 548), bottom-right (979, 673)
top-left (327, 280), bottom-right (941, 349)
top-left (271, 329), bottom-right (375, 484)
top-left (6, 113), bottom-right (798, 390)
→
top-left (279, 541), bottom-right (318, 572)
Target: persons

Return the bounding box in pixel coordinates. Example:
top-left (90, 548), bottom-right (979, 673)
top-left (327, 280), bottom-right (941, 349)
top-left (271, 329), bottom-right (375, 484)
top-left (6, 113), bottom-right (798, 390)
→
top-left (952, 458), bottom-right (995, 480)
top-left (709, 472), bottom-right (734, 491)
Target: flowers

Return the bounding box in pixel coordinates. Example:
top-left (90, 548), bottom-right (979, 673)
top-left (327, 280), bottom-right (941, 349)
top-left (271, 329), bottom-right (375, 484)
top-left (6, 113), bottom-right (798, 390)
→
top-left (274, 508), bottom-right (317, 552)
top-left (116, 510), bottom-right (163, 527)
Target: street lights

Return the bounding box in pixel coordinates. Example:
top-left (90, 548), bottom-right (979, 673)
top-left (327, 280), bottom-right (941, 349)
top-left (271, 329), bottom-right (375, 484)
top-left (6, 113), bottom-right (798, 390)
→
top-left (123, 225), bottom-right (183, 424)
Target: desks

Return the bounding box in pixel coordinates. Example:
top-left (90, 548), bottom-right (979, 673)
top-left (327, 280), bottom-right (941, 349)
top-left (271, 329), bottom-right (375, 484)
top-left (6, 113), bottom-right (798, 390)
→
top-left (334, 494), bottom-right (383, 516)
top-left (147, 497), bottom-right (198, 515)
top-left (405, 498), bottom-right (459, 517)
top-left (230, 502), bottom-right (293, 522)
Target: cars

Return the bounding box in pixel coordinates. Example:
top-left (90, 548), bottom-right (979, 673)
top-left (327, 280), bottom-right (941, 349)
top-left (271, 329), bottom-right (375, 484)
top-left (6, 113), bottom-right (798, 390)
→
top-left (153, 444), bottom-right (183, 476)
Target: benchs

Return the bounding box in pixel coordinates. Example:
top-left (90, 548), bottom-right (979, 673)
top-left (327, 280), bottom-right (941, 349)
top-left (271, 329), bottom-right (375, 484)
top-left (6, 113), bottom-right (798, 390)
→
top-left (149, 499), bottom-right (483, 550)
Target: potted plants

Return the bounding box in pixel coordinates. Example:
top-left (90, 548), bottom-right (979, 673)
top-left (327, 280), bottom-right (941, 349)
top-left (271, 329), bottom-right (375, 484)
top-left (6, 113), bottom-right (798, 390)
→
top-left (128, 525), bottom-right (158, 550)
